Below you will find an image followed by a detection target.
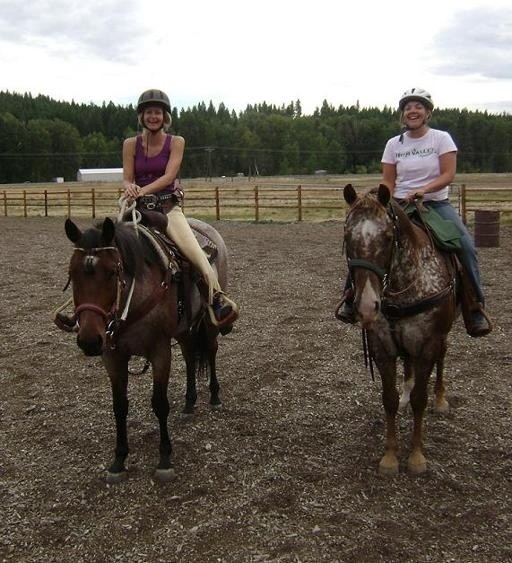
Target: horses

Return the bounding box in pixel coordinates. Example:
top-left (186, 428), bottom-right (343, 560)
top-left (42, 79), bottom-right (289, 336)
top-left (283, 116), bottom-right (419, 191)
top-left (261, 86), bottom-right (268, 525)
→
top-left (63, 217), bottom-right (229, 484)
top-left (342, 182), bottom-right (463, 477)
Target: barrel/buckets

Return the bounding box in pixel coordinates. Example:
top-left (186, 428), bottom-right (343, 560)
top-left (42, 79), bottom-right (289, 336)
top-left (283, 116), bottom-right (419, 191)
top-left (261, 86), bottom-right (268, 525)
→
top-left (474, 207), bottom-right (500, 247)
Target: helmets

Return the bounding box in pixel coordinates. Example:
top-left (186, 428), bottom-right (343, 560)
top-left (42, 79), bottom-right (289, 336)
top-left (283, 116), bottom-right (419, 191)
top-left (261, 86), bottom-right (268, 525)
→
top-left (138, 89), bottom-right (170, 112)
top-left (399, 87), bottom-right (433, 111)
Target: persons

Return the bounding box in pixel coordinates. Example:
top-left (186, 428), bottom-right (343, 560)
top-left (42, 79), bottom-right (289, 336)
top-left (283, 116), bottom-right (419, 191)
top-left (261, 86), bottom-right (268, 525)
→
top-left (56, 88), bottom-right (232, 322)
top-left (337, 87), bottom-right (486, 333)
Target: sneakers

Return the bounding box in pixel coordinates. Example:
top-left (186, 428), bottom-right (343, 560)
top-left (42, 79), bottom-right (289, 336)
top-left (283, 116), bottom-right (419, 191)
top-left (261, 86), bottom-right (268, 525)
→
top-left (57, 313), bottom-right (76, 326)
top-left (469, 312), bottom-right (489, 334)
top-left (216, 304), bottom-right (233, 334)
top-left (340, 302), bottom-right (354, 322)
